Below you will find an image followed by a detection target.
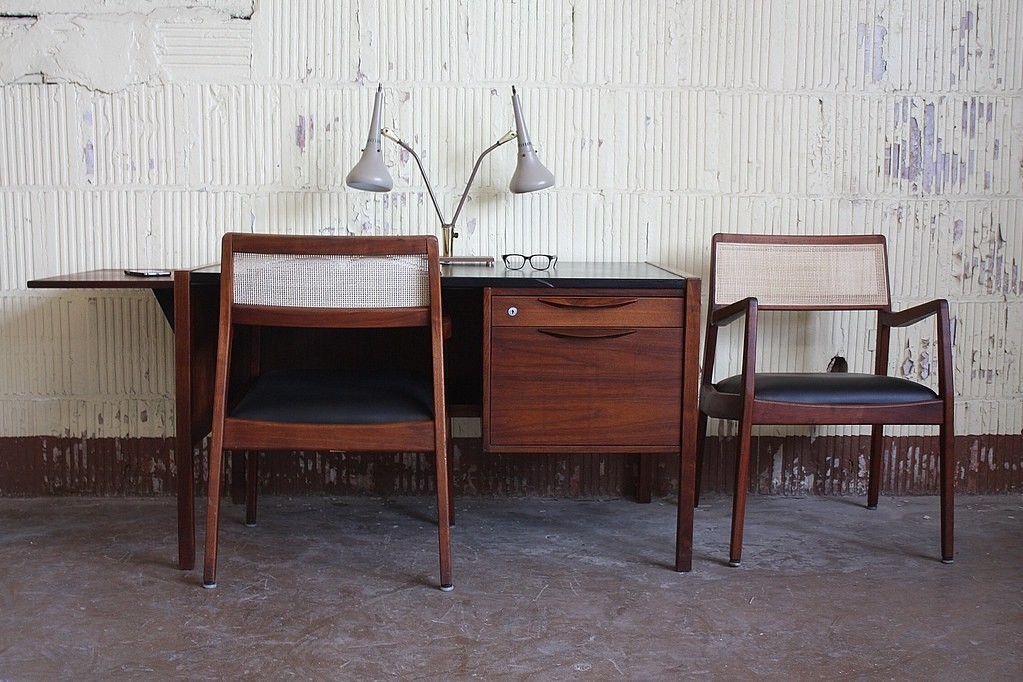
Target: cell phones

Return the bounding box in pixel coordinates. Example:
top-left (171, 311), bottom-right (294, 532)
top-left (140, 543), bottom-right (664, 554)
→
top-left (124, 269), bottom-right (172, 277)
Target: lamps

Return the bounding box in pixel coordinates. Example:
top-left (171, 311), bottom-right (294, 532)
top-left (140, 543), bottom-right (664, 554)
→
top-left (345, 83), bottom-right (556, 264)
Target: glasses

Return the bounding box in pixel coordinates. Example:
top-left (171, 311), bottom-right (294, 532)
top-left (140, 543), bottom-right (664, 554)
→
top-left (501, 253), bottom-right (558, 271)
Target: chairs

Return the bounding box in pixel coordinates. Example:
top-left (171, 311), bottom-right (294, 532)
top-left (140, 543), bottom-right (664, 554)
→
top-left (695, 233), bottom-right (957, 566)
top-left (201, 231), bottom-right (457, 593)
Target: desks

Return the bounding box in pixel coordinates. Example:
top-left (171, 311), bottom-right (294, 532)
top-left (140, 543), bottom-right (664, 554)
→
top-left (25, 268), bottom-right (175, 333)
top-left (173, 259), bottom-right (702, 573)
